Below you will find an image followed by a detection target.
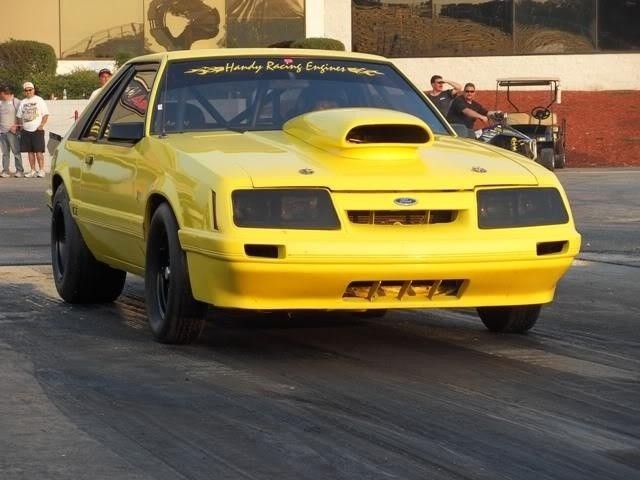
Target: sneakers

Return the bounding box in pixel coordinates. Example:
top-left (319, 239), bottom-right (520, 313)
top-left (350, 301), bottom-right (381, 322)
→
top-left (0, 170), bottom-right (45, 178)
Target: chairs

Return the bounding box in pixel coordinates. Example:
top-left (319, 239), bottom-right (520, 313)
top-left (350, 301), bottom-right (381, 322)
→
top-left (154, 101), bottom-right (204, 133)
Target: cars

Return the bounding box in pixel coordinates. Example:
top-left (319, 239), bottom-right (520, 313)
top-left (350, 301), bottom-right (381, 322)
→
top-left (46, 42), bottom-right (583, 348)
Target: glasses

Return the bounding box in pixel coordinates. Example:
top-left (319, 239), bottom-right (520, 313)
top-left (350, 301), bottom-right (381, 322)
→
top-left (464, 90), bottom-right (474, 93)
top-left (24, 88), bottom-right (34, 92)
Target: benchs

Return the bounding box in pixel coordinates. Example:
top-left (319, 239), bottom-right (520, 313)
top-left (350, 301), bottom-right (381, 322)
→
top-left (507, 112), bottom-right (557, 136)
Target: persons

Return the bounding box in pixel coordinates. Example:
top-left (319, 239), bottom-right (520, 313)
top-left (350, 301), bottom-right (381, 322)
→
top-left (15, 80), bottom-right (51, 178)
top-left (0, 85), bottom-right (25, 179)
top-left (427, 75), bottom-right (462, 120)
top-left (446, 82), bottom-right (508, 140)
top-left (86, 67), bottom-right (116, 103)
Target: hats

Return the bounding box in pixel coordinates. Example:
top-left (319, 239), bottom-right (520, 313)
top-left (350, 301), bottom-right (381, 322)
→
top-left (98, 68), bottom-right (112, 76)
top-left (22, 81), bottom-right (35, 90)
